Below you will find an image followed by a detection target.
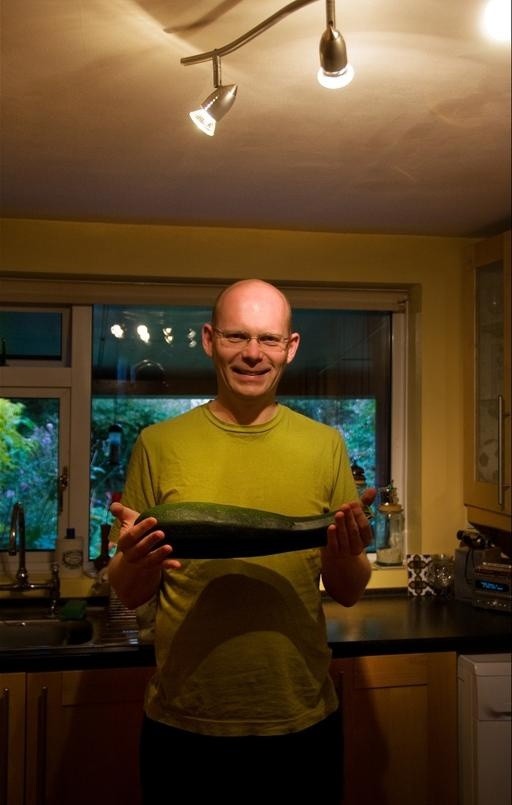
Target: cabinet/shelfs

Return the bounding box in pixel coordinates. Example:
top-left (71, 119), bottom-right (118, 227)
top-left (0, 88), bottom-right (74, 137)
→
top-left (0, 667), bottom-right (158, 805)
top-left (331, 652), bottom-right (458, 805)
top-left (465, 231), bottom-right (512, 533)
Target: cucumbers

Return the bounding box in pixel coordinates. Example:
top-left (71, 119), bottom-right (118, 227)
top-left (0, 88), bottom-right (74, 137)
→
top-left (133, 502), bottom-right (374, 559)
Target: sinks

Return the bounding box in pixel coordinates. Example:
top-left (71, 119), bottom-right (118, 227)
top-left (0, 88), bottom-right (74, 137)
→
top-left (0, 617), bottom-right (95, 673)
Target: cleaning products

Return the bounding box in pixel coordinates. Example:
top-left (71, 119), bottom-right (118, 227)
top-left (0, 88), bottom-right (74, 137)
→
top-left (56, 527), bottom-right (83, 580)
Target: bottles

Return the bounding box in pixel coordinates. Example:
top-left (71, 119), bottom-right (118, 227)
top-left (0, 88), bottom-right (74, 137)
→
top-left (431, 554), bottom-right (456, 598)
top-left (52, 526), bottom-right (85, 578)
top-left (350, 454), bottom-right (379, 572)
top-left (375, 480), bottom-right (407, 567)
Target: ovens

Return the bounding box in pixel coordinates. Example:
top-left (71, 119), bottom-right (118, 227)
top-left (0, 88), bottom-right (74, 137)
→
top-left (458, 650), bottom-right (512, 805)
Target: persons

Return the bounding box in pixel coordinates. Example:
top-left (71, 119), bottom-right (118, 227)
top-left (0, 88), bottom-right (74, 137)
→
top-left (107, 280), bottom-right (374, 802)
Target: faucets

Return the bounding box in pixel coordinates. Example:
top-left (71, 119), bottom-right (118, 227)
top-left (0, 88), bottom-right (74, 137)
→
top-left (8, 502), bottom-right (28, 583)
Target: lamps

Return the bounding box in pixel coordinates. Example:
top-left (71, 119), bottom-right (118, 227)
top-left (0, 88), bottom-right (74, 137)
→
top-left (112, 311), bottom-right (197, 348)
top-left (180, 1), bottom-right (355, 136)
